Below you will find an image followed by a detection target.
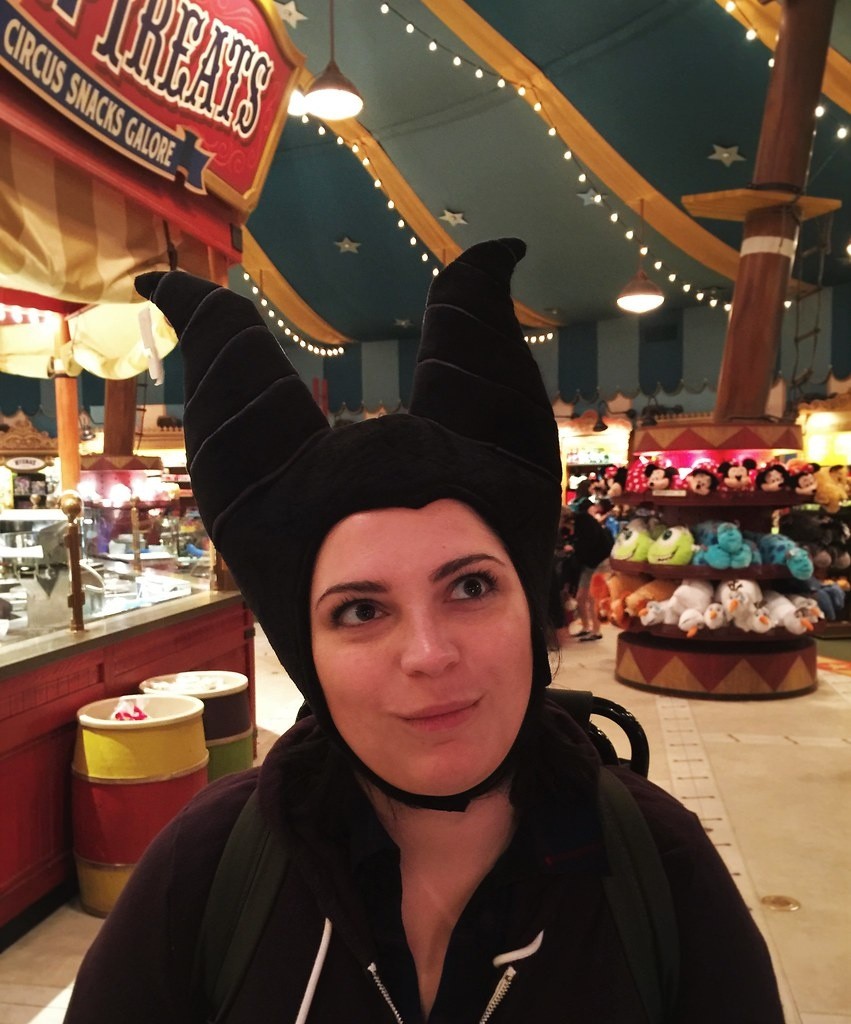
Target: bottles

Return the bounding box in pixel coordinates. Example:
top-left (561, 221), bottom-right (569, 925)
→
top-left (605, 517), bottom-right (619, 540)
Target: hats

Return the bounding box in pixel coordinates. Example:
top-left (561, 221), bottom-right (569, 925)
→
top-left (134, 237), bottom-right (564, 812)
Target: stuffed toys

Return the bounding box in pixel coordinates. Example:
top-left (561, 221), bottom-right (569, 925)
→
top-left (566, 458), bottom-right (851, 637)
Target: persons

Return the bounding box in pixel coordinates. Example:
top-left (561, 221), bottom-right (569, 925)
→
top-left (63, 238), bottom-right (786, 1023)
top-left (565, 479), bottom-right (615, 641)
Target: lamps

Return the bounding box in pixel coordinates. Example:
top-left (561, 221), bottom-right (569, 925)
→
top-left (617, 199), bottom-right (664, 313)
top-left (302, 1), bottom-right (363, 120)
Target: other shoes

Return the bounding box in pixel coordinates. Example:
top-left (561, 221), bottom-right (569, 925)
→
top-left (575, 631), bottom-right (590, 636)
top-left (579, 634), bottom-right (602, 642)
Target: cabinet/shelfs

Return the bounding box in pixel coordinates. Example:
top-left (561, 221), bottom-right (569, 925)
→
top-left (561, 452), bottom-right (850, 640)
